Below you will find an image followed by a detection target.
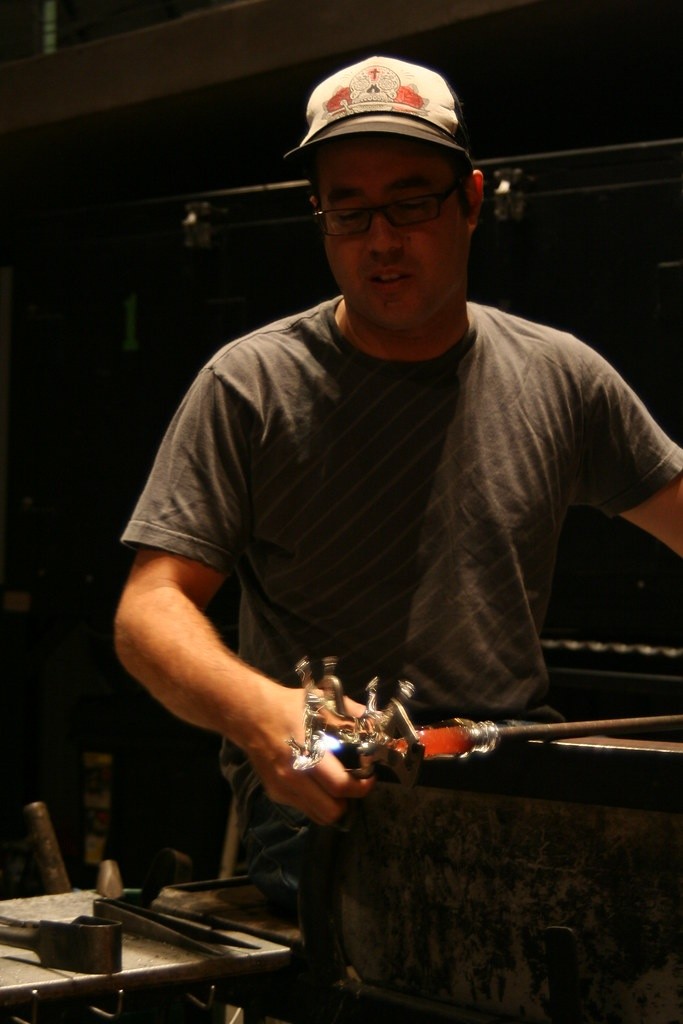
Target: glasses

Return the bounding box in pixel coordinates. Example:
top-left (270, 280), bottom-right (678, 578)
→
top-left (314, 179), bottom-right (461, 238)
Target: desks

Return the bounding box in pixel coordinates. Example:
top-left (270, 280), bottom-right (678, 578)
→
top-left (0, 888), bottom-right (295, 1024)
top-left (123, 876), bottom-right (308, 960)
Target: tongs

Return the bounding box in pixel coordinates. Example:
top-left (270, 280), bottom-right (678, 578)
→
top-left (0, 915), bottom-right (123, 974)
top-left (92, 898), bottom-right (260, 956)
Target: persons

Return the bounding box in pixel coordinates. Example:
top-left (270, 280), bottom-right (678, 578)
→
top-left (113, 56), bottom-right (683, 880)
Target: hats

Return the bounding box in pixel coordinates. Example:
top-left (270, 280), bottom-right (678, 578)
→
top-left (283, 55), bottom-right (475, 173)
